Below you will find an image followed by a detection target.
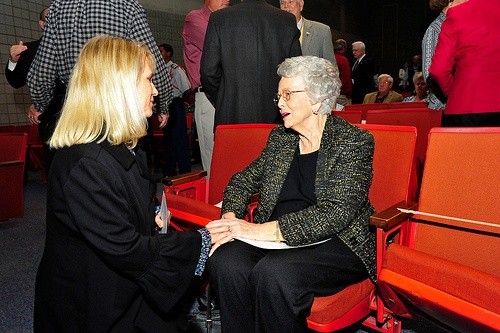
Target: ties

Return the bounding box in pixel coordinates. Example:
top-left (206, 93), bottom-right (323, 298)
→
top-left (353, 61), bottom-right (359, 71)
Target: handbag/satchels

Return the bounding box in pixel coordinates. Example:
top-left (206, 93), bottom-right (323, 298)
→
top-left (184, 281), bottom-right (223, 333)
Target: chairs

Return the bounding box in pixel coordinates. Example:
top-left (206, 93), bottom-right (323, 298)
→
top-left (0, 123), bottom-right (47, 227)
top-left (152, 92), bottom-right (500, 333)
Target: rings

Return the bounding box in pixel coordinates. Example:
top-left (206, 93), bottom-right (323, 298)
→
top-left (227, 226), bottom-right (230, 231)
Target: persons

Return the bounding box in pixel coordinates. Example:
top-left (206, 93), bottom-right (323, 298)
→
top-left (33, 35), bottom-right (235, 333)
top-left (200, 0), bottom-right (303, 133)
top-left (403, 72), bottom-right (430, 102)
top-left (26, 0), bottom-right (173, 128)
top-left (422, 0), bottom-right (469, 109)
top-left (182, 0), bottom-right (232, 178)
top-left (158, 44), bottom-right (192, 177)
top-left (428, 0), bottom-right (500, 128)
top-left (409, 54), bottom-right (422, 91)
top-left (280, 0), bottom-right (336, 65)
top-left (351, 42), bottom-right (376, 103)
top-left (399, 61), bottom-right (409, 93)
top-left (206, 55), bottom-right (394, 333)
top-left (4, 7), bottom-right (67, 148)
top-left (363, 74), bottom-right (403, 103)
top-left (334, 39), bottom-right (352, 97)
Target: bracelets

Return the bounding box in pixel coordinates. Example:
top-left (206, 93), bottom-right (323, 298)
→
top-left (276, 221), bottom-right (280, 243)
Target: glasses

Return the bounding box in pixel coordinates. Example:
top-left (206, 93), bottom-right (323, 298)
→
top-left (272, 89), bottom-right (305, 106)
